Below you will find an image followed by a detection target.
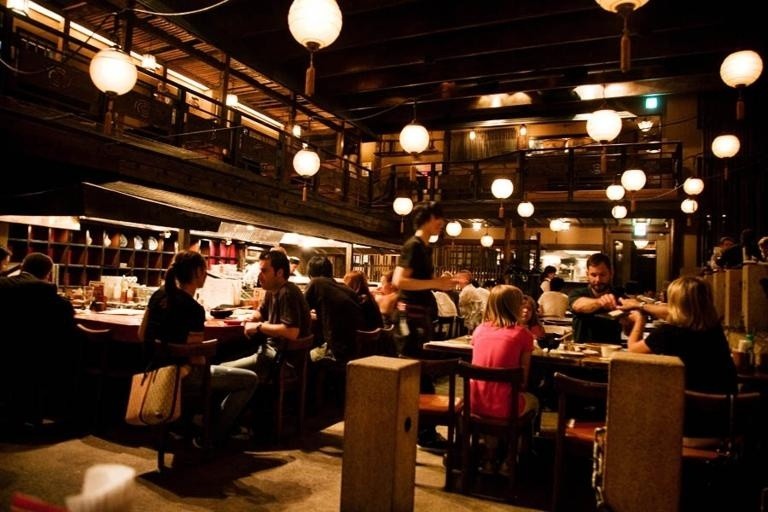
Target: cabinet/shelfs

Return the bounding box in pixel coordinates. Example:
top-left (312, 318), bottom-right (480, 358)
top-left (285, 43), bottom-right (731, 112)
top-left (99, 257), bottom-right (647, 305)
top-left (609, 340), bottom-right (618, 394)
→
top-left (0, 214), bottom-right (243, 290)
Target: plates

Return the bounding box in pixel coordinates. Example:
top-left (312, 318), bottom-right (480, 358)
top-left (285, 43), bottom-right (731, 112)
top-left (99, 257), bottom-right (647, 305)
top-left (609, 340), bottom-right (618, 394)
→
top-left (550, 342), bottom-right (607, 356)
top-left (134, 235), bottom-right (158, 251)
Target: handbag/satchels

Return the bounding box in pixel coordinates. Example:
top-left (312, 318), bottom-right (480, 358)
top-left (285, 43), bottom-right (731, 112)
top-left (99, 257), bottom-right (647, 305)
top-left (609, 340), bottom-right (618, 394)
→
top-left (124, 363), bottom-right (183, 426)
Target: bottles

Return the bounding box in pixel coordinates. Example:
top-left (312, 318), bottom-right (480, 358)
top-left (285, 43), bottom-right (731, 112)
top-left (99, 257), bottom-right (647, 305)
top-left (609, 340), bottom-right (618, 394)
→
top-left (86, 230), bottom-right (128, 248)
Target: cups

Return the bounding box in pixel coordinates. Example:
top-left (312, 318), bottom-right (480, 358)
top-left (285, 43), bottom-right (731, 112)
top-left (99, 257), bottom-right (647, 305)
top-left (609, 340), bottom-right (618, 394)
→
top-left (731, 348), bottom-right (754, 372)
top-left (58, 277), bottom-right (153, 312)
top-left (601, 344), bottom-right (622, 358)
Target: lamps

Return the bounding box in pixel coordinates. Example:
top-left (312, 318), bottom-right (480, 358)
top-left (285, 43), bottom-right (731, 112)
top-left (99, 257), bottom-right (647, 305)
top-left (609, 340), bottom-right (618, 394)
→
top-left (606, 174), bottom-right (624, 201)
top-left (399, 99), bottom-right (429, 180)
top-left (597, 0), bottom-right (649, 72)
top-left (720, 50), bottom-right (763, 119)
top-left (517, 192), bottom-right (534, 231)
top-left (620, 148), bottom-right (646, 212)
top-left (637, 119), bottom-right (654, 132)
top-left (90, 10), bottom-right (138, 133)
top-left (293, 123), bottom-right (302, 139)
top-left (491, 164), bottom-right (513, 218)
top-left (614, 218), bottom-right (652, 251)
top-left (683, 177), bottom-right (705, 196)
top-left (611, 205), bottom-right (627, 219)
top-left (520, 124), bottom-right (528, 136)
top-left (288, 0), bottom-right (342, 96)
top-left (393, 172), bottom-right (413, 233)
top-left (550, 220), bottom-right (570, 232)
top-left (142, 39), bottom-right (156, 70)
top-left (226, 80), bottom-right (237, 107)
top-left (428, 218), bottom-right (494, 250)
top-left (293, 119), bottom-right (320, 201)
top-left (681, 198), bottom-right (698, 226)
top-left (586, 62), bottom-right (622, 172)
top-left (711, 135), bottom-right (740, 179)
top-left (470, 129), bottom-right (476, 140)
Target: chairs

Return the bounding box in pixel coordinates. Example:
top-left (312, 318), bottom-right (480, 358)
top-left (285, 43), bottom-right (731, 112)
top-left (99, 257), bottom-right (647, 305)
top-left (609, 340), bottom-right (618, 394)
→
top-left (418, 314), bottom-right (768, 511)
top-left (3, 281), bottom-right (458, 475)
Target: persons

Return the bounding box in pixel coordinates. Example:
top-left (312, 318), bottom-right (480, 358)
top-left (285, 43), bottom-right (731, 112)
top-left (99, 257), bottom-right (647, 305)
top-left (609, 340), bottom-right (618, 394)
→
top-left (135, 249), bottom-right (258, 436)
top-left (0, 251), bottom-right (79, 423)
top-left (216, 245), bottom-right (303, 441)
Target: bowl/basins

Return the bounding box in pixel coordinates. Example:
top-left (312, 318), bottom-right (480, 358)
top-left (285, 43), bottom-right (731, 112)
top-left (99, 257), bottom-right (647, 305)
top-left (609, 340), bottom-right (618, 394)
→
top-left (210, 308), bottom-right (233, 319)
top-left (536, 336), bottom-right (561, 350)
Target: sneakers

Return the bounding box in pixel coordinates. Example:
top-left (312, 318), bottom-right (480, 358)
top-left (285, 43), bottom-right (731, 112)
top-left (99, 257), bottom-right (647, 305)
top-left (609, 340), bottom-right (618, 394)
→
top-left (417, 435), bottom-right (449, 453)
